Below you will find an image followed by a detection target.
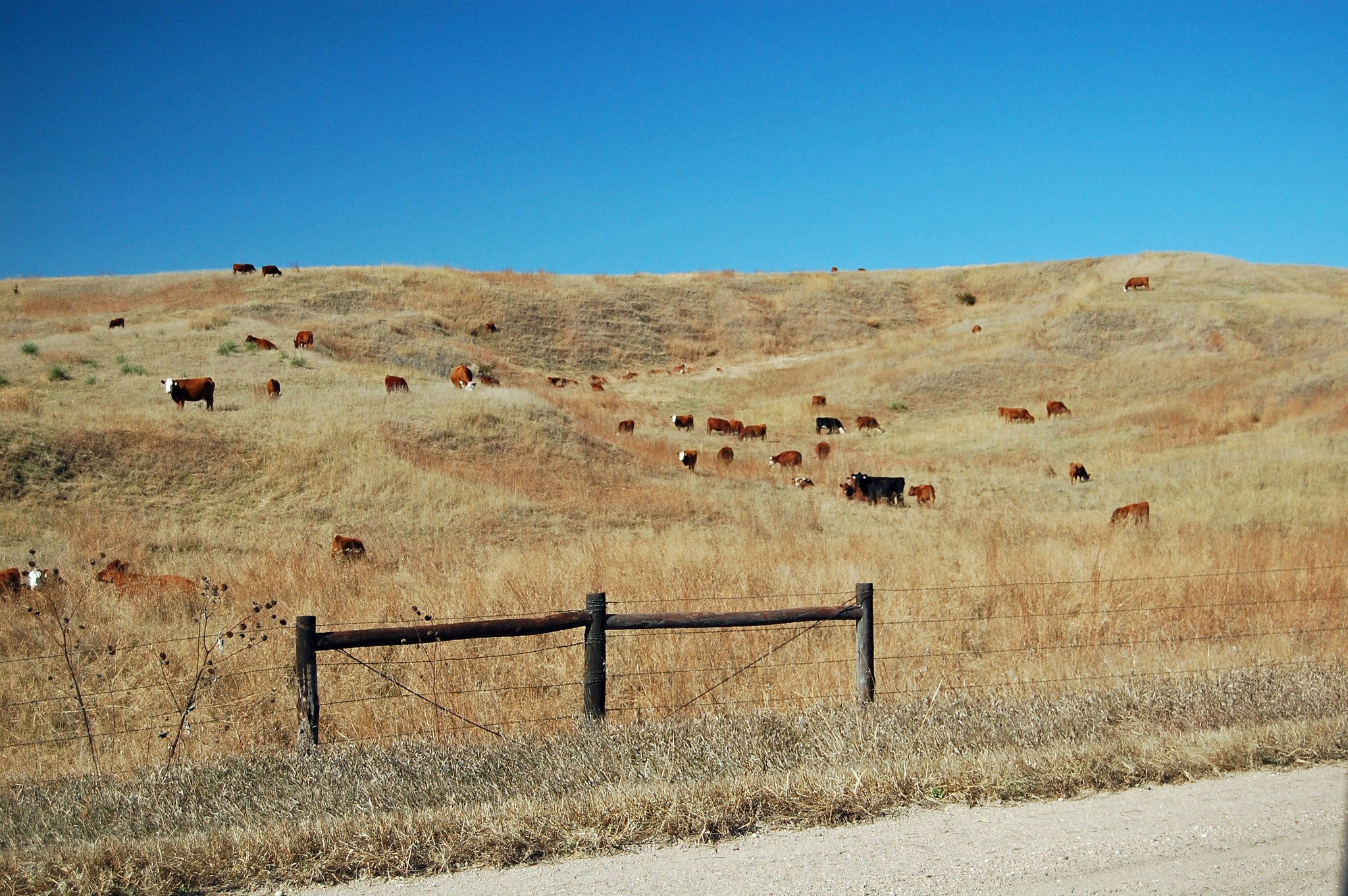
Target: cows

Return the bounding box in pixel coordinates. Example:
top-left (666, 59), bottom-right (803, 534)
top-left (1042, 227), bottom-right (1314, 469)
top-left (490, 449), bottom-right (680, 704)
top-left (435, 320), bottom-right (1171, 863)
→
top-left (716, 368), bottom-right (723, 373)
top-left (589, 374), bottom-right (610, 391)
top-left (244, 334), bottom-right (278, 351)
top-left (1107, 501), bottom-right (1149, 526)
top-left (544, 375), bottom-right (580, 390)
top-left (1045, 400), bottom-right (1071, 420)
top-left (107, 317), bottom-right (125, 329)
top-left (971, 324), bottom-right (983, 335)
top-left (485, 323), bottom-right (497, 334)
top-left (1067, 462), bottom-right (1092, 486)
top-left (1122, 275), bottom-right (1150, 292)
top-left (292, 330), bottom-right (315, 351)
top-left (0, 563), bottom-right (72, 604)
top-left (997, 407), bottom-right (1035, 425)
top-left (676, 449), bottom-right (698, 474)
top-left (614, 419), bottom-right (635, 436)
top-left (672, 362), bottom-right (694, 375)
top-left (709, 393), bottom-right (937, 507)
top-left (329, 534), bottom-right (368, 563)
top-left (831, 267), bottom-right (838, 273)
top-left (95, 558), bottom-right (201, 606)
top-left (266, 377), bottom-right (281, 400)
top-left (616, 371), bottom-right (639, 380)
top-left (450, 364), bottom-right (473, 390)
top-left (670, 414), bottom-right (695, 432)
top-left (477, 369), bottom-right (499, 385)
top-left (231, 263), bottom-right (282, 278)
top-left (159, 376), bottom-right (216, 413)
top-left (384, 374), bottom-right (410, 395)
top-left (856, 268), bottom-right (867, 272)
